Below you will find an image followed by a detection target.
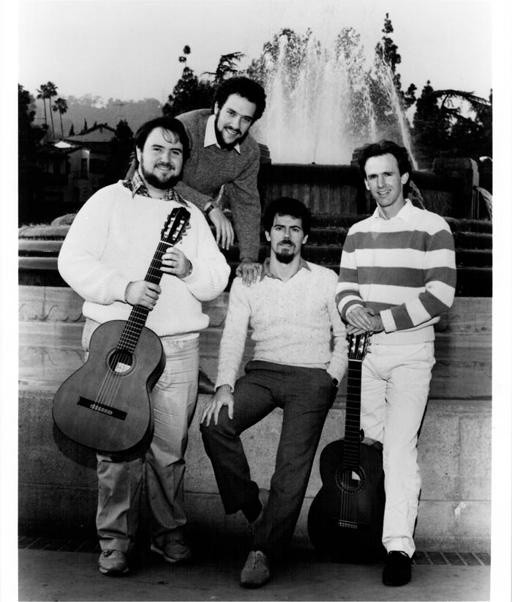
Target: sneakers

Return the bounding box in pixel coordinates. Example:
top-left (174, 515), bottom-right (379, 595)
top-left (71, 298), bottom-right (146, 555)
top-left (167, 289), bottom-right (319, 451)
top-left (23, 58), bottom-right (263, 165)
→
top-left (239, 481), bottom-right (262, 523)
top-left (151, 541), bottom-right (191, 562)
top-left (381, 551), bottom-right (411, 586)
top-left (240, 549), bottom-right (281, 588)
top-left (98, 550), bottom-right (129, 575)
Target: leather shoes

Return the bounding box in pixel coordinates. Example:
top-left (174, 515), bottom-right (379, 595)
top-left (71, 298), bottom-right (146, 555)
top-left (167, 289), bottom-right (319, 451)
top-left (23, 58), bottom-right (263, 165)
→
top-left (198, 370), bottom-right (215, 394)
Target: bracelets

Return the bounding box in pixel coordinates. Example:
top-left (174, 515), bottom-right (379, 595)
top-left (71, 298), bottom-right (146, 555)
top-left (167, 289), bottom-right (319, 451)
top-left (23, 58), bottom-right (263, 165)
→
top-left (188, 260), bottom-right (193, 274)
top-left (205, 202), bottom-right (219, 214)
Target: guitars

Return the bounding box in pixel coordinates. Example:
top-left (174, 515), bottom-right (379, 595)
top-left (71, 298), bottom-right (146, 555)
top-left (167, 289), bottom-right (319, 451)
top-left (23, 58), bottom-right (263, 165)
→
top-left (306, 329), bottom-right (381, 568)
top-left (51, 206), bottom-right (190, 458)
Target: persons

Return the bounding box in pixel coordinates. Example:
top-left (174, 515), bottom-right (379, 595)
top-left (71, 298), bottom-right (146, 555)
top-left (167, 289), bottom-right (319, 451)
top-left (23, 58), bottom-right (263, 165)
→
top-left (56, 116), bottom-right (233, 577)
top-left (335, 137), bottom-right (457, 587)
top-left (198, 197), bottom-right (349, 589)
top-left (124, 77), bottom-right (265, 288)
